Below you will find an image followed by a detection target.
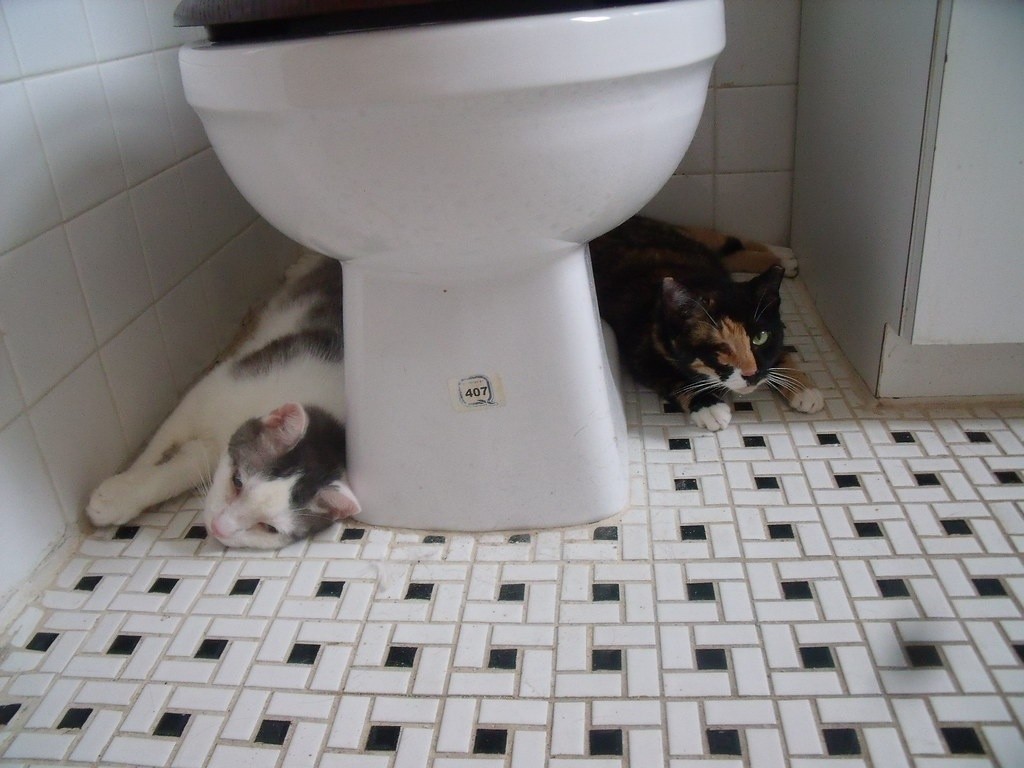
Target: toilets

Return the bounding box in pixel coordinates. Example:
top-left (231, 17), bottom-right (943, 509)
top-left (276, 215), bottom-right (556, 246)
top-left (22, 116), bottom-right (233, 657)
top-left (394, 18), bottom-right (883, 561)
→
top-left (170, 0), bottom-right (724, 531)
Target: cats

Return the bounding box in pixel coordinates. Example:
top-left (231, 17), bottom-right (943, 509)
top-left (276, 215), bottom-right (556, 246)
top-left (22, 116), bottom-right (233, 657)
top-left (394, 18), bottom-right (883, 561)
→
top-left (590, 215), bottom-right (828, 433)
top-left (83, 250), bottom-right (363, 567)
